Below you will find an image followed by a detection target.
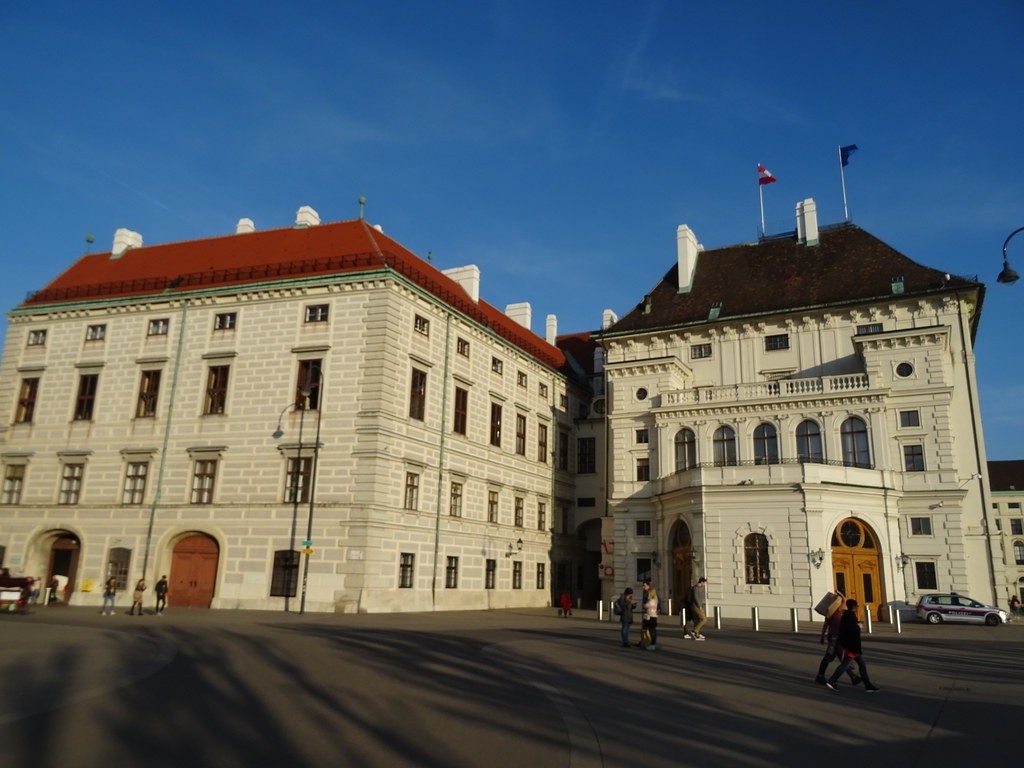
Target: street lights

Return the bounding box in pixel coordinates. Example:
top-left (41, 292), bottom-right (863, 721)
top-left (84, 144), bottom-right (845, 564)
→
top-left (298, 365), bottom-right (324, 615)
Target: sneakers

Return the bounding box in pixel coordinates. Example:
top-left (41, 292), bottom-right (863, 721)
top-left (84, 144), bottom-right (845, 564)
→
top-left (866, 684), bottom-right (880, 692)
top-left (826, 681), bottom-right (839, 691)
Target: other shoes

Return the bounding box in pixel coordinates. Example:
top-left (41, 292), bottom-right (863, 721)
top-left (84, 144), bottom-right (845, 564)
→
top-left (815, 676), bottom-right (827, 684)
top-left (853, 677), bottom-right (862, 686)
top-left (623, 643), bottom-right (631, 647)
top-left (645, 644), bottom-right (655, 651)
top-left (684, 632), bottom-right (706, 641)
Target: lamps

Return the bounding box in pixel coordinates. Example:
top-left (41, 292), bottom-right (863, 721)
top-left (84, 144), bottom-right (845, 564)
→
top-left (506, 538), bottom-right (523, 557)
top-left (690, 546), bottom-right (699, 562)
top-left (815, 547), bottom-right (825, 569)
top-left (651, 550), bottom-right (657, 563)
top-left (899, 554), bottom-right (910, 569)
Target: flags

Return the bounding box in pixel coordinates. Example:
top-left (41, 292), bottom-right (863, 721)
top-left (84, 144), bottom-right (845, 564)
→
top-left (758, 166), bottom-right (777, 184)
top-left (841, 145), bottom-right (858, 167)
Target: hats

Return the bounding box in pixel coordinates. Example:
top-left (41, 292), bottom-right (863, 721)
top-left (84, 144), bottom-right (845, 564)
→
top-left (624, 588), bottom-right (633, 595)
top-left (699, 577), bottom-right (706, 583)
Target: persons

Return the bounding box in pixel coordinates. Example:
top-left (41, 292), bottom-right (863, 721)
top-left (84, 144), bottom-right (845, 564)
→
top-left (639, 580), bottom-right (662, 650)
top-left (49, 575), bottom-right (58, 593)
top-left (101, 577), bottom-right (117, 616)
top-left (684, 578), bottom-right (708, 640)
top-left (155, 575), bottom-right (168, 616)
top-left (130, 579), bottom-right (148, 615)
top-left (31, 577), bottom-right (41, 605)
top-left (561, 589), bottom-right (571, 617)
top-left (618, 587), bottom-right (636, 647)
top-left (815, 590), bottom-right (880, 692)
top-left (1010, 595), bottom-right (1021, 620)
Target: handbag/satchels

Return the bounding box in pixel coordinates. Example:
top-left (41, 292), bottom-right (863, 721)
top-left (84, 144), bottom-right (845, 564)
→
top-left (643, 614), bottom-right (650, 621)
top-left (613, 601), bottom-right (622, 614)
top-left (814, 592), bottom-right (842, 618)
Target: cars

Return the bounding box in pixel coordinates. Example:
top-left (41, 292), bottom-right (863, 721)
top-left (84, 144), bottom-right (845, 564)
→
top-left (917, 592), bottom-right (1008, 625)
